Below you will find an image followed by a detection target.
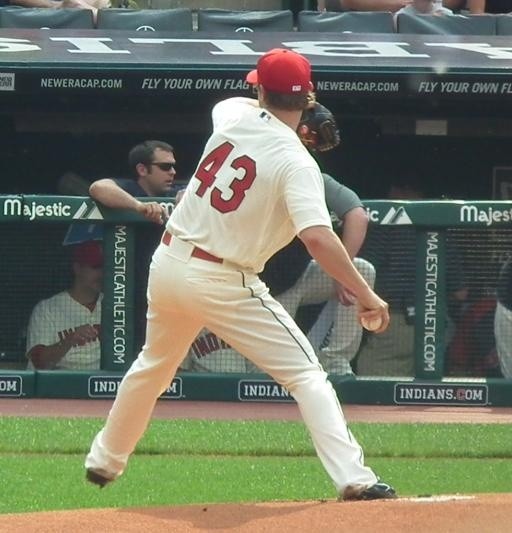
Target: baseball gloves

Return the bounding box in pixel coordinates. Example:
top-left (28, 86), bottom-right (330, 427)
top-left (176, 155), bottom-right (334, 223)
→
top-left (297, 102), bottom-right (340, 152)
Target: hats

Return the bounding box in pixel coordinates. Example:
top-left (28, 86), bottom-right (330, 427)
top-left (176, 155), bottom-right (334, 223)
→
top-left (70, 240), bottom-right (106, 268)
top-left (246, 48), bottom-right (312, 94)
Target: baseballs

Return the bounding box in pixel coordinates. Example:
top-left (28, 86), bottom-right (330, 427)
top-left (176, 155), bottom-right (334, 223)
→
top-left (361, 316), bottom-right (382, 331)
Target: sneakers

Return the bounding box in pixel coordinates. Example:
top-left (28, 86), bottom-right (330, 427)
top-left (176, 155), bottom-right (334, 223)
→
top-left (86, 468), bottom-right (111, 488)
top-left (318, 352), bottom-right (355, 377)
top-left (342, 480), bottom-right (398, 502)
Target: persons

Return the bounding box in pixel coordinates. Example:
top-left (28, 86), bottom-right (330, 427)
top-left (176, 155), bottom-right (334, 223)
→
top-left (86, 139), bottom-right (194, 371)
top-left (178, 329), bottom-right (248, 373)
top-left (262, 171), bottom-right (374, 377)
top-left (0, 0), bottom-right (512, 24)
top-left (26, 240), bottom-right (106, 373)
top-left (354, 182), bottom-right (511, 380)
top-left (81, 45), bottom-right (399, 500)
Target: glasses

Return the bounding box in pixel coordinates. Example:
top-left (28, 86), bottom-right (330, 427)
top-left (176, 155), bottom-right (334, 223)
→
top-left (142, 162), bottom-right (178, 170)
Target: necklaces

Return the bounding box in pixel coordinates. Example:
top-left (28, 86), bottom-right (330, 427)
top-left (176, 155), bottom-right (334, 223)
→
top-left (83, 301), bottom-right (96, 307)
